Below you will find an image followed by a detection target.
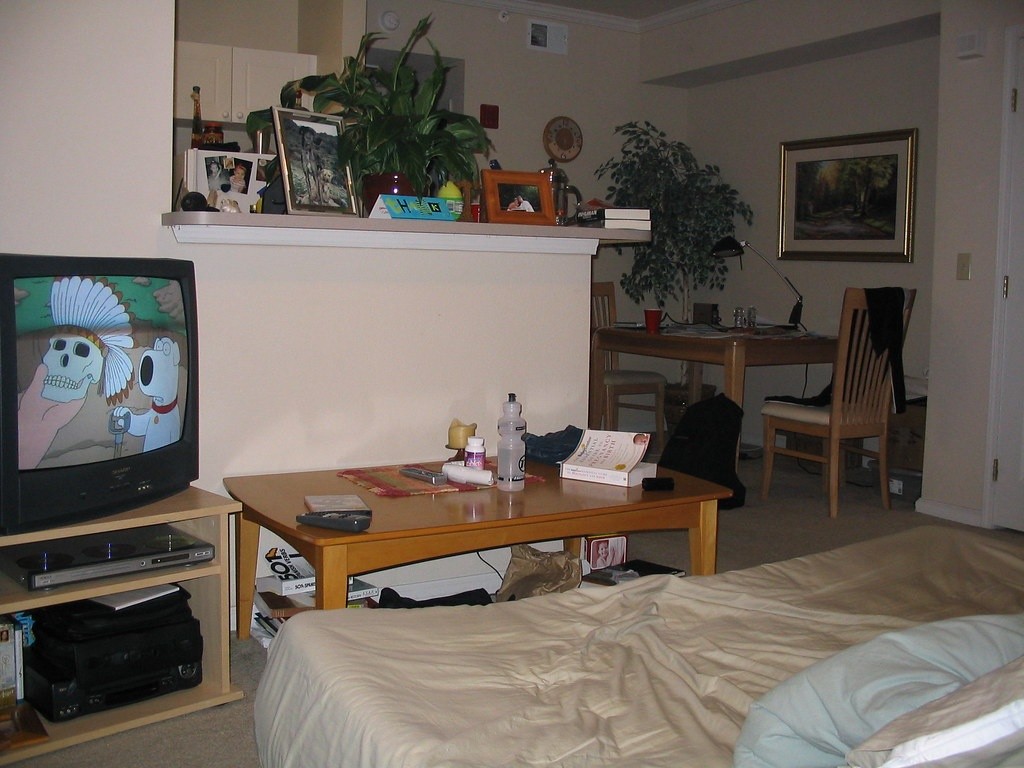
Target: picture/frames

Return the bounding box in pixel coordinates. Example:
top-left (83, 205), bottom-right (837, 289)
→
top-left (271, 105), bottom-right (360, 219)
top-left (776, 128), bottom-right (919, 263)
top-left (480, 169), bottom-right (557, 226)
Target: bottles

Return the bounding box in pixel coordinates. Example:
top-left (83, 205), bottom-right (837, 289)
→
top-left (747, 306), bottom-right (757, 329)
top-left (495, 393), bottom-right (527, 492)
top-left (463, 435), bottom-right (486, 471)
top-left (734, 307), bottom-right (745, 328)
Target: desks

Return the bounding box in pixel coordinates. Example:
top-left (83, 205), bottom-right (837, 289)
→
top-left (223, 451), bottom-right (733, 642)
top-left (591, 325), bottom-right (840, 480)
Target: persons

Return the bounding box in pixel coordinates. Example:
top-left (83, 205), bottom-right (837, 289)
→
top-left (207, 161), bottom-right (222, 190)
top-left (633, 434), bottom-right (649, 445)
top-left (230, 166), bottom-right (246, 194)
top-left (517, 195), bottom-right (534, 212)
top-left (597, 541), bottom-right (615, 568)
top-left (507, 198), bottom-right (519, 211)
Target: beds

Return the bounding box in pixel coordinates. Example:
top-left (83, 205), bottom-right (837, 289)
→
top-left (252, 526), bottom-right (1024, 767)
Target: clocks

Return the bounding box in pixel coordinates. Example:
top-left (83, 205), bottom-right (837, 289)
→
top-left (543, 116), bottom-right (583, 163)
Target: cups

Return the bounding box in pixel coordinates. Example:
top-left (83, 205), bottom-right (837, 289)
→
top-left (644, 309), bottom-right (661, 336)
top-left (538, 158), bottom-right (582, 226)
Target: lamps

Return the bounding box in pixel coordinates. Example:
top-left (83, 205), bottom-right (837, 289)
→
top-left (709, 236), bottom-right (807, 333)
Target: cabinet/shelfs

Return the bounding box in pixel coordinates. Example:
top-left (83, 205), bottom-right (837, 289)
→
top-left (174, 40), bottom-right (317, 124)
top-left (1, 484), bottom-right (244, 766)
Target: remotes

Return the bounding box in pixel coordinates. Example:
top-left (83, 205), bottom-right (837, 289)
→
top-left (399, 467), bottom-right (448, 486)
top-left (296, 511), bottom-right (371, 532)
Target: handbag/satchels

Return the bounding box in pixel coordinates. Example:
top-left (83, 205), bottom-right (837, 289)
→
top-left (11, 581), bottom-right (205, 723)
top-left (519, 425), bottom-right (591, 466)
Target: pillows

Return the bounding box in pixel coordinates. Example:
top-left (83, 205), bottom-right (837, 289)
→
top-left (843, 653), bottom-right (1024, 768)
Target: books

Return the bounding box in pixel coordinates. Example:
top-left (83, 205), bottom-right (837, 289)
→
top-left (578, 208), bottom-right (651, 231)
top-left (582, 559), bottom-right (687, 586)
top-left (89, 583), bottom-right (180, 611)
top-left (559, 429), bottom-right (657, 488)
top-left (253, 547), bottom-right (379, 639)
top-left (0, 614), bottom-right (24, 711)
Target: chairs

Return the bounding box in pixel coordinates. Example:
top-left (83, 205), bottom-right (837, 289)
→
top-left (591, 281), bottom-right (666, 457)
top-left (761, 286), bottom-right (917, 520)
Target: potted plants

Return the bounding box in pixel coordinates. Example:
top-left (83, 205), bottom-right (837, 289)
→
top-left (594, 120), bottom-right (754, 433)
top-left (245, 12), bottom-right (496, 218)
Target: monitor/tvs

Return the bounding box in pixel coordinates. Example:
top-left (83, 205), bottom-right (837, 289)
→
top-left (0, 252), bottom-right (200, 539)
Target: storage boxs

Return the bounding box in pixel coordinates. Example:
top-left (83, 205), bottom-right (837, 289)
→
top-left (584, 534), bottom-right (629, 571)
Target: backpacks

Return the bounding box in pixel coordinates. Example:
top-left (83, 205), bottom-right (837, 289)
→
top-left (659, 393), bottom-right (747, 510)
